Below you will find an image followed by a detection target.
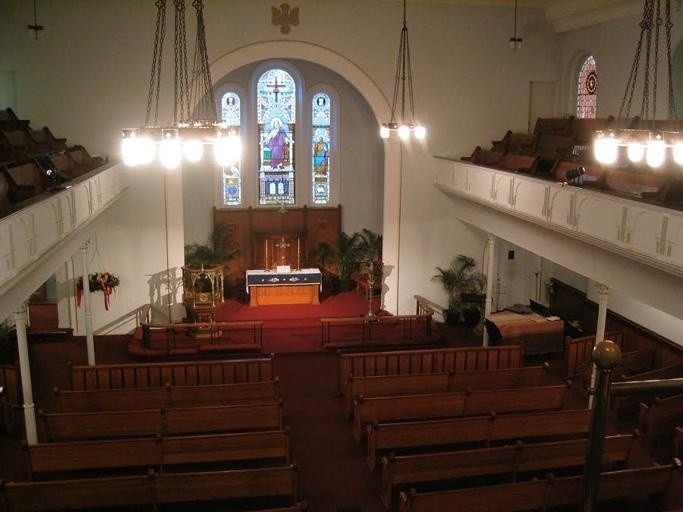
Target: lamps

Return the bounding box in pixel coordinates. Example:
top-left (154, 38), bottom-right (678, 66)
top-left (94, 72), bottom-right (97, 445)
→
top-left (379, 1), bottom-right (430, 141)
top-left (119, 0), bottom-right (245, 170)
top-left (592, 0), bottom-right (683, 169)
top-left (509, 0), bottom-right (522, 53)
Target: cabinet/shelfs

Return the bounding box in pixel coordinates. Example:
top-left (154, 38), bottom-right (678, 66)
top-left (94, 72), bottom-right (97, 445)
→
top-left (245, 269), bottom-right (322, 306)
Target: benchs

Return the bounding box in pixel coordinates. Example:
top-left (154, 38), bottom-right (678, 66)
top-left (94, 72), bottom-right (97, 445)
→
top-left (460, 117), bottom-right (683, 212)
top-left (1, 107), bottom-right (105, 220)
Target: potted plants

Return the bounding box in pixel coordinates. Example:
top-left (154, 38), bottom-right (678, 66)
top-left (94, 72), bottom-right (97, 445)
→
top-left (459, 272), bottom-right (488, 327)
top-left (429, 254), bottom-right (474, 324)
